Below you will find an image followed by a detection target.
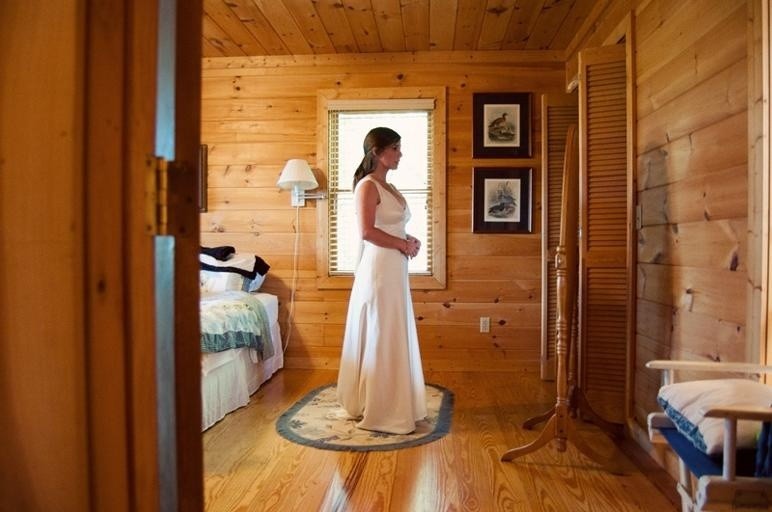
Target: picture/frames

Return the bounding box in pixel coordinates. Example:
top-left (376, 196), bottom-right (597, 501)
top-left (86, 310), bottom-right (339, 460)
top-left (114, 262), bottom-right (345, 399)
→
top-left (470, 166), bottom-right (534, 235)
top-left (198, 143), bottom-right (208, 212)
top-left (471, 90), bottom-right (533, 158)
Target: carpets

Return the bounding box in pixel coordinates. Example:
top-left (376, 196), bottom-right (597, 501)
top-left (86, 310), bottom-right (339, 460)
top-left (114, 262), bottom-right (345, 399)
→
top-left (275, 381), bottom-right (453, 453)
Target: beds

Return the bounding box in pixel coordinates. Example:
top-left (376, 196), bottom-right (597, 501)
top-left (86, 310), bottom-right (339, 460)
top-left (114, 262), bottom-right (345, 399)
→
top-left (199, 244), bottom-right (286, 435)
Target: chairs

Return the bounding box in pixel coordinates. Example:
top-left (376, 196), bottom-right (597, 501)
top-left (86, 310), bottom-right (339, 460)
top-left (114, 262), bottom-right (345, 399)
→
top-left (644, 360), bottom-right (772, 512)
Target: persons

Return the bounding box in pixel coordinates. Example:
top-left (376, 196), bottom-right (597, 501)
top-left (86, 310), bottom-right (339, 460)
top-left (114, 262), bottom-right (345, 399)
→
top-left (335, 126), bottom-right (430, 435)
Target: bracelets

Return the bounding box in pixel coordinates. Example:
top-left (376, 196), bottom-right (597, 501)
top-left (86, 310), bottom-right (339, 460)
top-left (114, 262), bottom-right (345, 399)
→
top-left (400, 239), bottom-right (408, 253)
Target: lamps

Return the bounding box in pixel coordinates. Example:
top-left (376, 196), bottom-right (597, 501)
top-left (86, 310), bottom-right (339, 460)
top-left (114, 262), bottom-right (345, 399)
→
top-left (278, 159), bottom-right (328, 206)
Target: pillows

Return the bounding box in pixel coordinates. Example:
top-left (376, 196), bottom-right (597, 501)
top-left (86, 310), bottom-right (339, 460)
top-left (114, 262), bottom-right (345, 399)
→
top-left (244, 272), bottom-right (266, 293)
top-left (199, 268), bottom-right (251, 293)
top-left (657, 377), bottom-right (772, 456)
